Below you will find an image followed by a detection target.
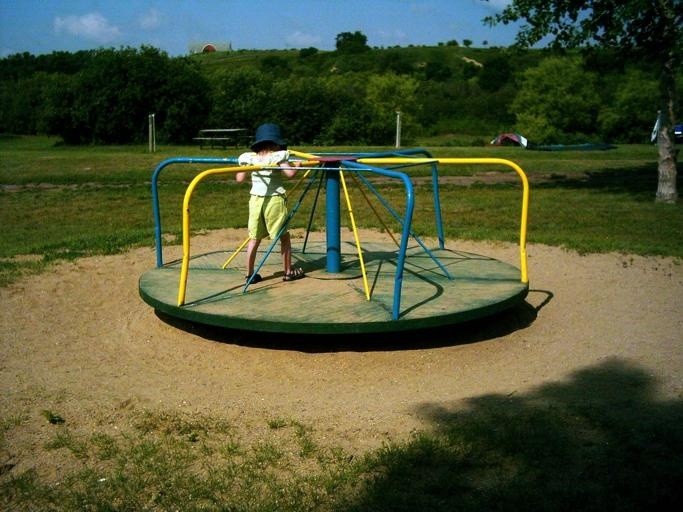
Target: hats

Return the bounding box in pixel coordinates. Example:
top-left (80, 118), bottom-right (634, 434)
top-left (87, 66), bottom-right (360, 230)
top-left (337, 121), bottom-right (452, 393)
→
top-left (249, 124), bottom-right (288, 150)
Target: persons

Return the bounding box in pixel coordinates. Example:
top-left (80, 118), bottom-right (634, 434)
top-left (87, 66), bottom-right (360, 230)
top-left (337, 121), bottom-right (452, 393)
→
top-left (236, 123), bottom-right (305, 284)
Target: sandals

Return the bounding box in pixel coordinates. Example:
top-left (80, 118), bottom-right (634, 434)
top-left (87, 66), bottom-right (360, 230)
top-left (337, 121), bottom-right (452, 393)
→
top-left (244, 273), bottom-right (261, 284)
top-left (283, 267), bottom-right (305, 282)
